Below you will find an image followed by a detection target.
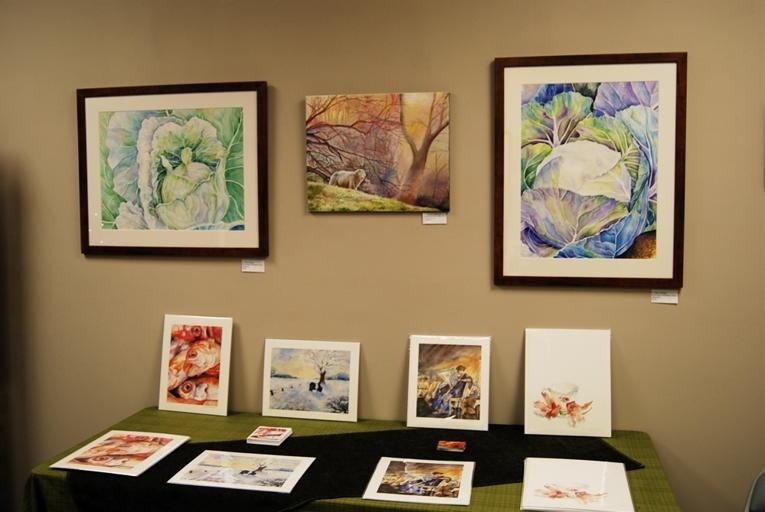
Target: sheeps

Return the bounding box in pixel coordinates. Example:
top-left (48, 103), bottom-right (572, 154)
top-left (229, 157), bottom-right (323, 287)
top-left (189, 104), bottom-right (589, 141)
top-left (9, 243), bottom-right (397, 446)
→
top-left (328, 169), bottom-right (366, 190)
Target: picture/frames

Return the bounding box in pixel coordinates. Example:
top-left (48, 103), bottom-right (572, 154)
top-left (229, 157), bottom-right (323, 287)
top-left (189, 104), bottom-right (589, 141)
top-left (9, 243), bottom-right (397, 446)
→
top-left (75, 80), bottom-right (271, 262)
top-left (489, 49), bottom-right (689, 291)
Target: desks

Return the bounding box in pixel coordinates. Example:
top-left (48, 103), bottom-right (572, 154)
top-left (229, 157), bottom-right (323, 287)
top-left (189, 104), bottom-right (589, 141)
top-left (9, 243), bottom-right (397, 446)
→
top-left (30, 405), bottom-right (683, 511)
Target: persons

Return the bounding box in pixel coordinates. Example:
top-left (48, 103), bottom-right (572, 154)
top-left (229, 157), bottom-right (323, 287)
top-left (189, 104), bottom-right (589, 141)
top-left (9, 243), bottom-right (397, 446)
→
top-left (405, 472), bottom-right (448, 493)
top-left (434, 365), bottom-right (471, 415)
